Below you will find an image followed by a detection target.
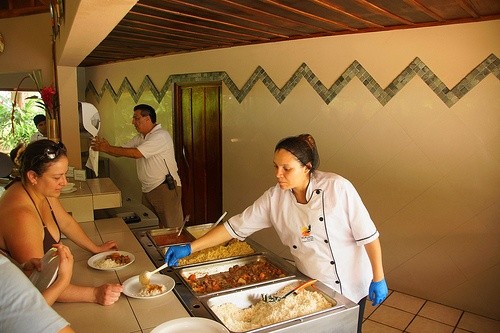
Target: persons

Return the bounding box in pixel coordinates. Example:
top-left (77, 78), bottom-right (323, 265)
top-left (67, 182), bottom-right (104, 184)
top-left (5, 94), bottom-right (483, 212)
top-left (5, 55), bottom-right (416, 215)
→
top-left (0, 139), bottom-right (123, 305)
top-left (90, 104), bottom-right (184, 228)
top-left (166, 134), bottom-right (389, 333)
top-left (0, 242), bottom-right (75, 333)
top-left (30, 114), bottom-right (47, 141)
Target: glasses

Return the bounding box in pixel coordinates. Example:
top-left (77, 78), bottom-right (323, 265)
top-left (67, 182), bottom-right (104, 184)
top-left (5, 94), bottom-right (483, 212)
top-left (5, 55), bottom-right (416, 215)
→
top-left (38, 141), bottom-right (68, 162)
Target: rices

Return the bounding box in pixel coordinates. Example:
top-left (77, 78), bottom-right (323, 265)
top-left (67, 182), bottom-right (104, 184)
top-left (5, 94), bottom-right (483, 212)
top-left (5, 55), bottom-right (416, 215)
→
top-left (138, 284), bottom-right (165, 296)
top-left (211, 285), bottom-right (331, 331)
top-left (94, 259), bottom-right (121, 268)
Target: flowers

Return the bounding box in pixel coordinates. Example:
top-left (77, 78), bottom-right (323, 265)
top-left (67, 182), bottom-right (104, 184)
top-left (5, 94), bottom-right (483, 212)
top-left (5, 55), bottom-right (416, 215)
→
top-left (10, 70), bottom-right (59, 136)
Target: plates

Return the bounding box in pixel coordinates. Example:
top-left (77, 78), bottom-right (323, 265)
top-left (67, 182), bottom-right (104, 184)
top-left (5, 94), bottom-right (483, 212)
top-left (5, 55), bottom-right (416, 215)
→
top-left (87, 251), bottom-right (135, 271)
top-left (60, 187), bottom-right (77, 194)
top-left (149, 317), bottom-right (230, 333)
top-left (61, 183), bottom-right (74, 192)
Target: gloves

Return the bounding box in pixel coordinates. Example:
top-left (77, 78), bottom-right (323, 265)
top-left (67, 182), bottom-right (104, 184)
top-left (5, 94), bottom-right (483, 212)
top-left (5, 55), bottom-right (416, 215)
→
top-left (369, 280), bottom-right (388, 306)
top-left (165, 244), bottom-right (191, 267)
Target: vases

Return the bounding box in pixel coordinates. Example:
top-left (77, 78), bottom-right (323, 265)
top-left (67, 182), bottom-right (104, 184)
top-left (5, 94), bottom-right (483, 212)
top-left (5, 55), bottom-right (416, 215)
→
top-left (47, 118), bottom-right (59, 141)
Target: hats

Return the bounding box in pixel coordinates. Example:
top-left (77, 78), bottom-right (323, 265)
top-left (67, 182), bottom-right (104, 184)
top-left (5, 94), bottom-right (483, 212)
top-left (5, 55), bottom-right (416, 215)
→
top-left (34, 114), bottom-right (46, 126)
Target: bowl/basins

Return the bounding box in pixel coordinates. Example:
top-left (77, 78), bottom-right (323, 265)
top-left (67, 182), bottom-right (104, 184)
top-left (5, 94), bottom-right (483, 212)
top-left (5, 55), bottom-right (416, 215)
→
top-left (122, 273), bottom-right (176, 299)
